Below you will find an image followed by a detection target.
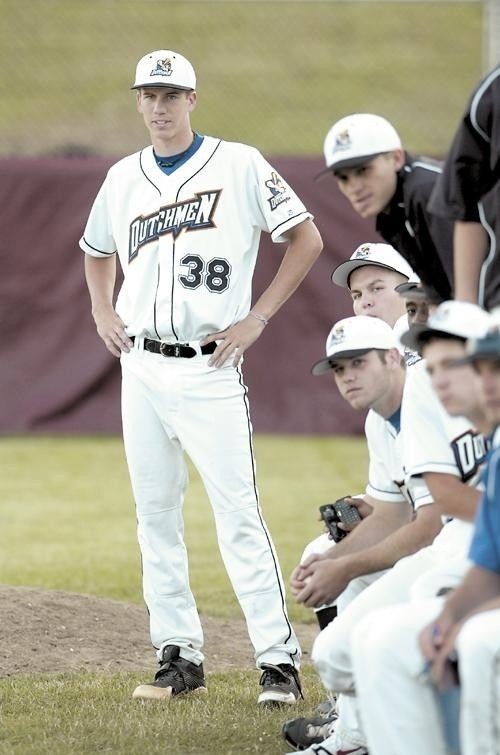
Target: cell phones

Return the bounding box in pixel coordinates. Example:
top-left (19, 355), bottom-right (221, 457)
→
top-left (333, 495), bottom-right (361, 523)
top-left (319, 504), bottom-right (348, 544)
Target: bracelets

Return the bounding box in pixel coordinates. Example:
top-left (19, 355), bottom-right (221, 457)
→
top-left (248, 312), bottom-right (268, 326)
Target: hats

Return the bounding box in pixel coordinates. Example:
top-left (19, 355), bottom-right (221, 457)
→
top-left (399, 301), bottom-right (500, 368)
top-left (132, 49), bottom-right (197, 96)
top-left (330, 242), bottom-right (430, 304)
top-left (311, 112), bottom-right (403, 187)
top-left (311, 314), bottom-right (401, 376)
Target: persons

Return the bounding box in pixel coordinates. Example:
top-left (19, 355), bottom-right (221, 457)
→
top-left (289, 63), bottom-right (500, 754)
top-left (75, 50), bottom-right (324, 704)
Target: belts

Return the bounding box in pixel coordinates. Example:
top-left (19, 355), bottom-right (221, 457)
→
top-left (126, 336), bottom-right (219, 359)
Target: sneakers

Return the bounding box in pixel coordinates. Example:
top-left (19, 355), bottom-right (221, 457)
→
top-left (279, 691), bottom-right (369, 754)
top-left (131, 644), bottom-right (206, 701)
top-left (256, 660), bottom-right (304, 708)
